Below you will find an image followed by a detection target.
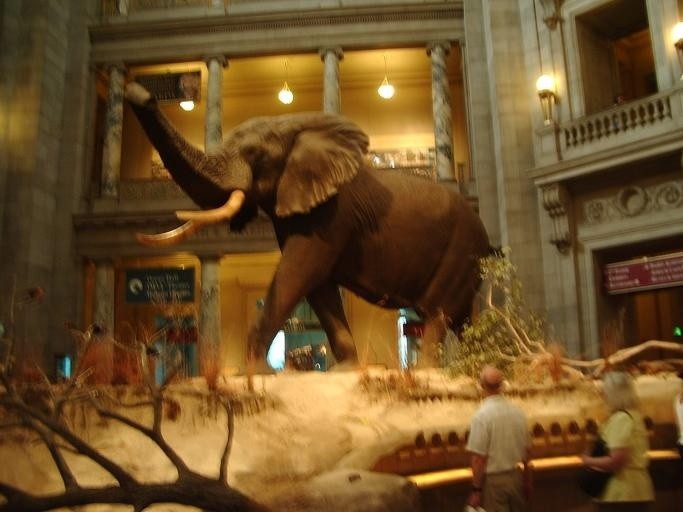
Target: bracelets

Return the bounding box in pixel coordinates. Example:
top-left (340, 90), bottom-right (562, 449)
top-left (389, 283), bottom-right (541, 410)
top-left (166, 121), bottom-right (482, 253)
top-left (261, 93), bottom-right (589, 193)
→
top-left (472, 485), bottom-right (481, 491)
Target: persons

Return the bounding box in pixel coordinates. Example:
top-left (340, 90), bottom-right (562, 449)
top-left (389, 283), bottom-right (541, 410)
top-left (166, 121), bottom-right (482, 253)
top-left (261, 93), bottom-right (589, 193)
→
top-left (462, 362), bottom-right (536, 512)
top-left (669, 366), bottom-right (682, 462)
top-left (578, 366), bottom-right (657, 511)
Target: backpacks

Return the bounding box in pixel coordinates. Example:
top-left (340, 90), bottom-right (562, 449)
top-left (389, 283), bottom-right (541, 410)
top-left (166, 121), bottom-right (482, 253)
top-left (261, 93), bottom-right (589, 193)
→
top-left (578, 408), bottom-right (634, 499)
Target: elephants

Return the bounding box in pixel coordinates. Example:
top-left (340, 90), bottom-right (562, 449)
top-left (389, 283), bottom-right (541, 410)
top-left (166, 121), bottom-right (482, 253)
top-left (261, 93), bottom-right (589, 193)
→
top-left (122, 80), bottom-right (506, 378)
